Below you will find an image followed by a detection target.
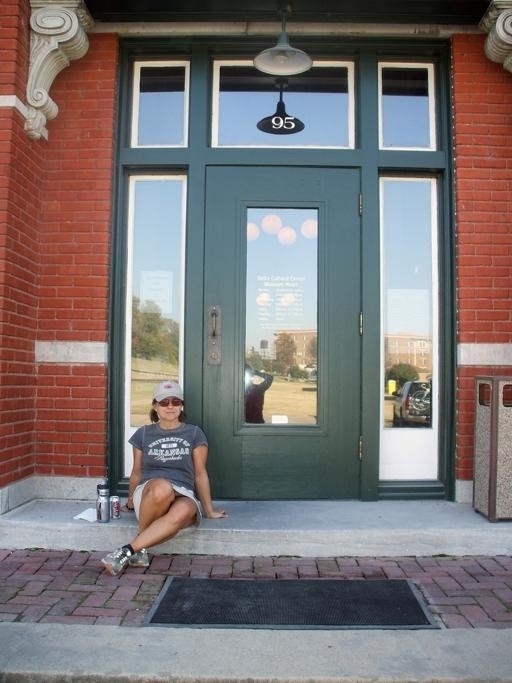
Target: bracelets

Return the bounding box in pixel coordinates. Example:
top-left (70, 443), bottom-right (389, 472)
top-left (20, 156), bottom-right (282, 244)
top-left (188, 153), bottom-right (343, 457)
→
top-left (125, 502), bottom-right (134, 510)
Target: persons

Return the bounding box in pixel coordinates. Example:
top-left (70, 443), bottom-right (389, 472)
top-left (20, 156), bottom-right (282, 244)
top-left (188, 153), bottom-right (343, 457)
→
top-left (100, 379), bottom-right (230, 576)
top-left (243, 359), bottom-right (273, 423)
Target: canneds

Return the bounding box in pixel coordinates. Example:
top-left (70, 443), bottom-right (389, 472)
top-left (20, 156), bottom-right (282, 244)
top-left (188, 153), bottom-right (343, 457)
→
top-left (109, 496), bottom-right (121, 521)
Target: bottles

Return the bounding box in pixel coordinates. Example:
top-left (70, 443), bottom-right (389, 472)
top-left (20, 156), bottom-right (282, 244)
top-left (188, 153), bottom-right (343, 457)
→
top-left (97, 483), bottom-right (110, 523)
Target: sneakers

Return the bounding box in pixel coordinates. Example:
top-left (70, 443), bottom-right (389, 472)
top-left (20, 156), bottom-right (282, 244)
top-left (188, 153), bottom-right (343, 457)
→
top-left (128, 547), bottom-right (149, 566)
top-left (100, 546), bottom-right (132, 575)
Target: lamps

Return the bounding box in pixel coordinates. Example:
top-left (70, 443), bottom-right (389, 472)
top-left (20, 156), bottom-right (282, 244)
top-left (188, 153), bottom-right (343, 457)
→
top-left (253, 11), bottom-right (312, 77)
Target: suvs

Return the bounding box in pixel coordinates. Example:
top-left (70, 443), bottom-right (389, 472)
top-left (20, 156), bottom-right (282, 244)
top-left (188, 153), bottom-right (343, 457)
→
top-left (392, 380), bottom-right (431, 425)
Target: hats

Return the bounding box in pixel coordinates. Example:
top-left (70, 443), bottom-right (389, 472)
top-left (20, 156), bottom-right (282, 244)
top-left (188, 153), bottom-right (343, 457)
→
top-left (153, 379), bottom-right (183, 402)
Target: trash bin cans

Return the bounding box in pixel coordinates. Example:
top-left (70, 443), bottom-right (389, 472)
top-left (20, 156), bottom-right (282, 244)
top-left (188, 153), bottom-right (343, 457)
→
top-left (472, 375), bottom-right (512, 522)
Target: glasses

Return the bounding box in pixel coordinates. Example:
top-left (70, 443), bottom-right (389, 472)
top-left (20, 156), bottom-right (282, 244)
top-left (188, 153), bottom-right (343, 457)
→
top-left (158, 398), bottom-right (182, 406)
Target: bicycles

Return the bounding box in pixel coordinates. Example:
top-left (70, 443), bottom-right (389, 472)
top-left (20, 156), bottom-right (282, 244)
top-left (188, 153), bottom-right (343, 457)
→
top-left (412, 383), bottom-right (430, 411)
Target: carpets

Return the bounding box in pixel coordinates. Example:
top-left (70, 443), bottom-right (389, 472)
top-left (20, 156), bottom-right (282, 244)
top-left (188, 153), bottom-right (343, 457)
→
top-left (140, 576), bottom-right (441, 630)
